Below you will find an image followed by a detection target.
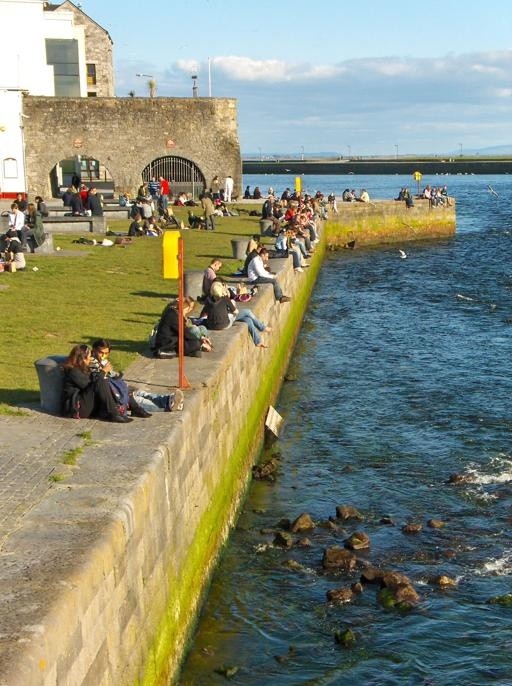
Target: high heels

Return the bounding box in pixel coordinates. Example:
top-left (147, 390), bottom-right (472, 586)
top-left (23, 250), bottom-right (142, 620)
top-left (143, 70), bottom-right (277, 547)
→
top-left (202, 338), bottom-right (211, 345)
top-left (200, 343), bottom-right (212, 352)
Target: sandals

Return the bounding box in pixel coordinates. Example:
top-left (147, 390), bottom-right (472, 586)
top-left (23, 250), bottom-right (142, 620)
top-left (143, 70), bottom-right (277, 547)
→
top-left (263, 328), bottom-right (272, 332)
top-left (256, 344), bottom-right (269, 348)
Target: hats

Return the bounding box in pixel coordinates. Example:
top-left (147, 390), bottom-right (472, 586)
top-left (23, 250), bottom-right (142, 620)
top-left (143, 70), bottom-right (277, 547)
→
top-left (280, 227), bottom-right (286, 233)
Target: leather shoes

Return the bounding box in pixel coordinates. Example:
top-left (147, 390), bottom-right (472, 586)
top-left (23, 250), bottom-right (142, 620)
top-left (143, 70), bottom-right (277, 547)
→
top-left (131, 409), bottom-right (153, 418)
top-left (309, 249), bottom-right (315, 252)
top-left (279, 297), bottom-right (290, 303)
top-left (109, 414), bottom-right (134, 424)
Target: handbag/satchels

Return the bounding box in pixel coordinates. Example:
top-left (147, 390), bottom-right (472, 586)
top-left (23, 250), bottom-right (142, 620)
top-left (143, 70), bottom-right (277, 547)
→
top-left (108, 375), bottom-right (129, 405)
top-left (115, 237), bottom-right (124, 244)
top-left (148, 329), bottom-right (157, 350)
top-left (236, 284), bottom-right (246, 294)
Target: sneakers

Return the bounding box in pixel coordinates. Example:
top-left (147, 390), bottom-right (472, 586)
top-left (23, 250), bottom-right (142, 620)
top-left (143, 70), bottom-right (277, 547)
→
top-left (294, 267), bottom-right (304, 273)
top-left (315, 232), bottom-right (318, 236)
top-left (301, 264), bottom-right (310, 267)
top-left (168, 393), bottom-right (174, 411)
top-left (251, 287), bottom-right (258, 296)
top-left (304, 255), bottom-right (312, 259)
top-left (313, 240), bottom-right (318, 243)
top-left (174, 389), bottom-right (184, 411)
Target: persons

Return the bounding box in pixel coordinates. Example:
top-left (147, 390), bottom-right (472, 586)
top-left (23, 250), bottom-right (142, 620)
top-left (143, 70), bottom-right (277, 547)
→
top-left (245, 185), bottom-right (250, 198)
top-left (143, 216), bottom-right (163, 235)
top-left (80, 186), bottom-right (88, 205)
top-left (88, 339), bottom-right (184, 412)
top-left (187, 192), bottom-right (192, 201)
top-left (62, 188), bottom-right (75, 210)
top-left (425, 185), bottom-right (436, 208)
top-left (432, 187), bottom-right (444, 208)
top-left (89, 171), bottom-right (92, 181)
top-left (184, 296), bottom-right (211, 352)
top-left (280, 219), bottom-right (289, 227)
top-left (205, 281), bottom-right (239, 330)
top-left (246, 234), bottom-right (260, 255)
top-left (351, 190), bottom-right (356, 196)
top-left (275, 229), bottom-right (304, 273)
top-left (199, 193), bottom-right (215, 230)
top-left (179, 192), bottom-right (187, 204)
top-left (360, 189), bottom-right (369, 202)
top-left (306, 210), bottom-right (319, 237)
top-left (24, 203), bottom-right (45, 253)
top-left (202, 258), bottom-right (258, 298)
top-left (300, 216), bottom-right (319, 244)
top-left (72, 172), bottom-right (80, 188)
top-left (200, 277), bottom-right (272, 348)
top-left (131, 203), bottom-right (140, 220)
top-left (343, 189), bottom-right (353, 202)
top-left (274, 188), bottom-right (328, 220)
top-left (35, 196), bottom-right (48, 218)
top-left (188, 210), bottom-right (202, 229)
top-left (287, 221), bottom-right (311, 259)
top-left (138, 183), bottom-right (149, 197)
top-left (223, 175), bottom-right (234, 203)
top-left (222, 205), bottom-right (227, 213)
top-left (91, 186), bottom-right (104, 207)
top-left (1, 234), bottom-right (21, 253)
top-left (69, 193), bottom-right (82, 216)
top-left (212, 175), bottom-right (220, 199)
top-left (129, 215), bottom-right (144, 237)
top-left (77, 183), bottom-right (89, 193)
top-left (404, 189), bottom-right (414, 207)
top-left (289, 231), bottom-right (310, 268)
top-left (157, 301), bottom-right (203, 358)
top-left (437, 186), bottom-right (449, 207)
top-left (149, 178), bottom-right (157, 194)
top-left (123, 193), bottom-right (129, 202)
top-left (12, 192), bottom-right (29, 215)
top-left (394, 187), bottom-right (405, 201)
top-left (262, 195), bottom-right (279, 237)
top-left (254, 187), bottom-right (260, 198)
top-left (158, 200), bottom-right (164, 217)
top-left (268, 188), bottom-right (275, 198)
top-left (329, 193), bottom-right (335, 212)
top-left (6, 203), bottom-right (24, 240)
top-left (243, 243), bottom-right (271, 275)
top-left (296, 215), bottom-right (316, 253)
top-left (71, 185), bottom-right (77, 193)
top-left (87, 191), bottom-right (103, 217)
top-left (159, 176), bottom-right (169, 209)
top-left (61, 344), bottom-right (152, 423)
top-left (442, 185), bottom-right (453, 206)
top-left (248, 248), bottom-right (292, 303)
